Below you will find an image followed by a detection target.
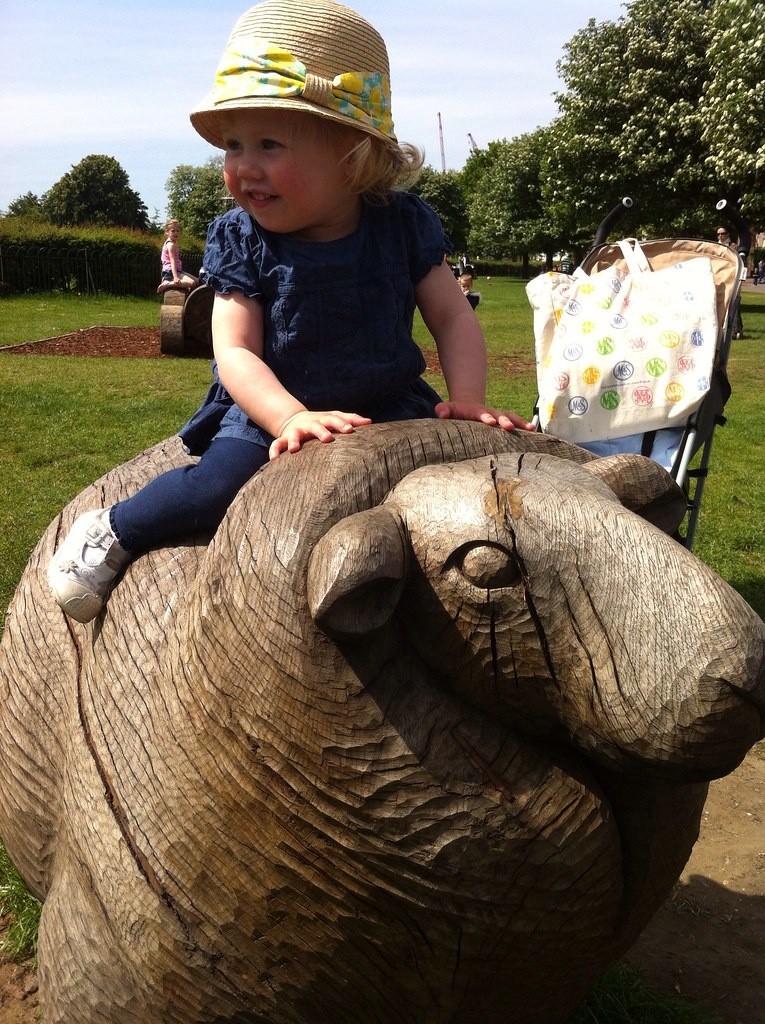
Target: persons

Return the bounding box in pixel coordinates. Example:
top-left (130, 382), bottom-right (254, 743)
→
top-left (158, 219), bottom-right (199, 293)
top-left (715, 227), bottom-right (765, 341)
top-left (47, 1), bottom-right (537, 626)
top-left (455, 252), bottom-right (481, 309)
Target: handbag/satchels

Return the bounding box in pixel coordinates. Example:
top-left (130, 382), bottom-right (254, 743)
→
top-left (525, 237), bottom-right (720, 444)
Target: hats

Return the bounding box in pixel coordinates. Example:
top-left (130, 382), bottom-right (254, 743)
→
top-left (190, 1), bottom-right (398, 151)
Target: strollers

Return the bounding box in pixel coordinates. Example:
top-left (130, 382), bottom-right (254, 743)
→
top-left (524, 195), bottom-right (754, 553)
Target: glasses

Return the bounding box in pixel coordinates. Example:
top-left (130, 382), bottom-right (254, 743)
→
top-left (717, 232), bottom-right (728, 237)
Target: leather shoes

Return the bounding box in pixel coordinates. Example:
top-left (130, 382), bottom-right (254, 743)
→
top-left (47, 511), bottom-right (129, 622)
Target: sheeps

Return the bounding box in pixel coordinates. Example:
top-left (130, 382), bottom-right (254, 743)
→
top-left (2, 417), bottom-right (763, 1022)
